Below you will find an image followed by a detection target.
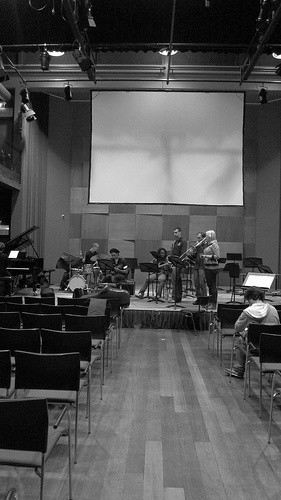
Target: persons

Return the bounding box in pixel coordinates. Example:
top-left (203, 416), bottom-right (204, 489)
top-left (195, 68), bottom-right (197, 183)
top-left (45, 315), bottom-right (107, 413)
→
top-left (223, 286), bottom-right (281, 378)
top-left (0, 241), bottom-right (8, 269)
top-left (186, 232), bottom-right (209, 305)
top-left (135, 249), bottom-right (171, 301)
top-left (199, 230), bottom-right (221, 309)
top-left (102, 249), bottom-right (130, 283)
top-left (83, 243), bottom-right (100, 268)
top-left (169, 227), bottom-right (187, 303)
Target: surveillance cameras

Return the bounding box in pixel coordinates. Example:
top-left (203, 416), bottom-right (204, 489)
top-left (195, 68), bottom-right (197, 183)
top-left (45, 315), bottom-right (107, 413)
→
top-left (25, 110), bottom-right (36, 121)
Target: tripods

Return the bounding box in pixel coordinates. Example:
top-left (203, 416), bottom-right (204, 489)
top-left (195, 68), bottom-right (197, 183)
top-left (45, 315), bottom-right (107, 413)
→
top-left (141, 251), bottom-right (273, 308)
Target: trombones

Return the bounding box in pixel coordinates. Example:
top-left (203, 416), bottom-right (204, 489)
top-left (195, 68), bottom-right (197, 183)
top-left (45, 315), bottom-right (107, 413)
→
top-left (179, 236), bottom-right (207, 261)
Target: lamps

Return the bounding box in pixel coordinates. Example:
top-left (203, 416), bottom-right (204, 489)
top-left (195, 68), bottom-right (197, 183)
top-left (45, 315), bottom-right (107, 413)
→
top-left (0, 48), bottom-right (281, 123)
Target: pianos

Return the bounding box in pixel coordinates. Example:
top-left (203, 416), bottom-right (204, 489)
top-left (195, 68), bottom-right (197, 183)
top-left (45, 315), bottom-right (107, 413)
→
top-left (0, 225), bottom-right (44, 291)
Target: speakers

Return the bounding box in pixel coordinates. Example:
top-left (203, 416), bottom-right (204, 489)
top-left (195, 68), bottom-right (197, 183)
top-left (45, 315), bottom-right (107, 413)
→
top-left (116, 292), bottom-right (132, 306)
top-left (121, 280), bottom-right (136, 295)
top-left (41, 288), bottom-right (55, 297)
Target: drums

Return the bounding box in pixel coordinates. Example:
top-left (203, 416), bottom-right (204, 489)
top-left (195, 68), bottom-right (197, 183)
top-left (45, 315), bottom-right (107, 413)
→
top-left (68, 274), bottom-right (86, 292)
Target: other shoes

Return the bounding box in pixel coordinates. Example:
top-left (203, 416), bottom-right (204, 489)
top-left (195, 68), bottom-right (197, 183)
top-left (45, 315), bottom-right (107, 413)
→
top-left (193, 300), bottom-right (198, 305)
top-left (135, 292), bottom-right (143, 298)
top-left (171, 300), bottom-right (175, 303)
top-left (157, 294), bottom-right (160, 298)
top-left (224, 367), bottom-right (244, 379)
top-left (202, 302), bottom-right (216, 309)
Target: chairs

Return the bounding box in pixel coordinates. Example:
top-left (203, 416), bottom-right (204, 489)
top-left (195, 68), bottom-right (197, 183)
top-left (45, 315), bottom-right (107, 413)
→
top-left (0, 297), bottom-right (123, 500)
top-left (147, 268), bottom-right (193, 299)
top-left (207, 304), bottom-right (281, 444)
